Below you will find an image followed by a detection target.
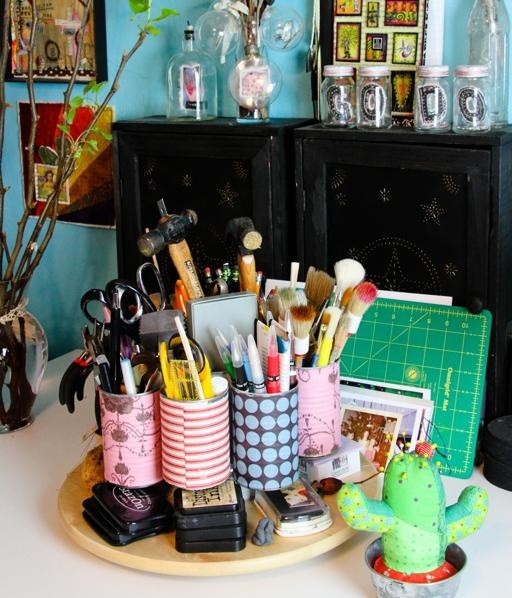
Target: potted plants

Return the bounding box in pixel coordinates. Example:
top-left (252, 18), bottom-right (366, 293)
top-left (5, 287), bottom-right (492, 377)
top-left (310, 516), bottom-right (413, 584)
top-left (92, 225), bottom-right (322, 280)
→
top-left (2, 0), bottom-right (184, 436)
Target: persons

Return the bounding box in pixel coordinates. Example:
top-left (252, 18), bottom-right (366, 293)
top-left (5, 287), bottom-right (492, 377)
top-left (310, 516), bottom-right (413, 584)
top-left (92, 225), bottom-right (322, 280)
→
top-left (42, 169), bottom-right (55, 189)
top-left (359, 428), bottom-right (369, 454)
top-left (364, 437), bottom-right (376, 462)
top-left (347, 429), bottom-right (357, 442)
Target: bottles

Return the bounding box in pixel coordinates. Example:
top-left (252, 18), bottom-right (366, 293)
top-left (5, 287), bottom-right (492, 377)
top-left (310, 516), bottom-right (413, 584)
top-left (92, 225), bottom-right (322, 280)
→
top-left (413, 65), bottom-right (453, 132)
top-left (320, 63), bottom-right (358, 129)
top-left (164, 25), bottom-right (219, 122)
top-left (453, 64), bottom-right (492, 136)
top-left (355, 65), bottom-right (393, 131)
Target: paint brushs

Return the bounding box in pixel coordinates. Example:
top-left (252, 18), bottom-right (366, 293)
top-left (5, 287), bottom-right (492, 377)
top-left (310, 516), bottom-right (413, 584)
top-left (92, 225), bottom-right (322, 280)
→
top-left (262, 258), bottom-right (377, 366)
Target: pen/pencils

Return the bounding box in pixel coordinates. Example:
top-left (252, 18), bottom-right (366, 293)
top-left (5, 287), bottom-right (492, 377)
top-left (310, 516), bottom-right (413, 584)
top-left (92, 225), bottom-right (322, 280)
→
top-left (204, 263), bottom-right (263, 297)
top-left (215, 310), bottom-right (292, 396)
top-left (81, 291), bottom-right (121, 393)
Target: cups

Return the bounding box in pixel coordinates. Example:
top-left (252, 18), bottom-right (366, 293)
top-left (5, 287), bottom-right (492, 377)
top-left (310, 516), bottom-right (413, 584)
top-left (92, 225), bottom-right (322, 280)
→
top-left (98, 355), bottom-right (341, 493)
top-left (365, 538), bottom-right (467, 598)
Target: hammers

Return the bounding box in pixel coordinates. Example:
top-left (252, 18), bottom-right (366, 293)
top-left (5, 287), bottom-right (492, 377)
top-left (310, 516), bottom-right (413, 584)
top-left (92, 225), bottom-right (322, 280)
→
top-left (136, 209), bottom-right (201, 299)
top-left (224, 213), bottom-right (263, 291)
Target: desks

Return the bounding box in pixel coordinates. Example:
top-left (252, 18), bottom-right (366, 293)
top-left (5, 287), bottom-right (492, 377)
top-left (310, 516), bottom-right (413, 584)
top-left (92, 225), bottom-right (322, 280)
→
top-left (0, 346), bottom-right (512, 598)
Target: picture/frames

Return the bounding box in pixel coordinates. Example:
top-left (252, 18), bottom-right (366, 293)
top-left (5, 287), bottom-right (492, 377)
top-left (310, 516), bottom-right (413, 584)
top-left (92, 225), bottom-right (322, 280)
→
top-left (3, 2), bottom-right (108, 85)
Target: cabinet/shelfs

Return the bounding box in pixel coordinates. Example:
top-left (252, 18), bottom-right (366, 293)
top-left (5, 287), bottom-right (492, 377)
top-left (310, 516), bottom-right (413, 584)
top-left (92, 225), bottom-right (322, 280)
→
top-left (108, 111), bottom-right (512, 462)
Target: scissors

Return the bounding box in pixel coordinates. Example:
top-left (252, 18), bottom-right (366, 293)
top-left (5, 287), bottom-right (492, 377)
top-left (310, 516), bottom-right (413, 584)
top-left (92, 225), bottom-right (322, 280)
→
top-left (131, 333), bottom-right (206, 400)
top-left (81, 263), bottom-right (168, 348)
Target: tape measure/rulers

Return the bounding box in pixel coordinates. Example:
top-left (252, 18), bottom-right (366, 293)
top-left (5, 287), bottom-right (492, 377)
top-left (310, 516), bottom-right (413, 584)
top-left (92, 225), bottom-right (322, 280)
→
top-left (170, 360), bottom-right (200, 400)
